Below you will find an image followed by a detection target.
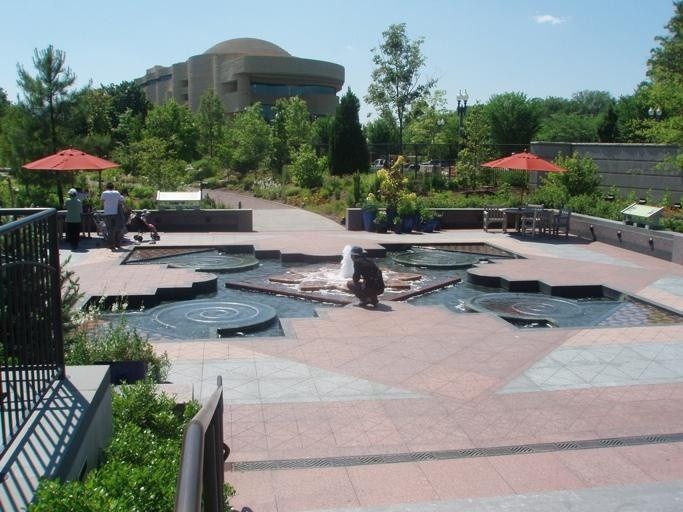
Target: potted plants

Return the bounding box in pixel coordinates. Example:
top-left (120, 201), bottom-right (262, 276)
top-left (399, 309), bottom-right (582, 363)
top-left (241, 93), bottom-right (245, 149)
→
top-left (91, 296), bottom-right (171, 386)
top-left (360, 171), bottom-right (444, 234)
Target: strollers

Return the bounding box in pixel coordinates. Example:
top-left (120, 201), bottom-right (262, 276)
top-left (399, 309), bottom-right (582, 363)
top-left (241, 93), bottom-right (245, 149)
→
top-left (123, 210), bottom-right (161, 242)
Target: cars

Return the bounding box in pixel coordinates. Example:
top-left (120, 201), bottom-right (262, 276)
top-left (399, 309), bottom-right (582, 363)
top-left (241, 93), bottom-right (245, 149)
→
top-left (370, 158), bottom-right (387, 168)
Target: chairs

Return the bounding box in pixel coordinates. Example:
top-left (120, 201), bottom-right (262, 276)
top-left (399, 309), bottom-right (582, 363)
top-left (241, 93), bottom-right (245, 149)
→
top-left (481, 203), bottom-right (572, 242)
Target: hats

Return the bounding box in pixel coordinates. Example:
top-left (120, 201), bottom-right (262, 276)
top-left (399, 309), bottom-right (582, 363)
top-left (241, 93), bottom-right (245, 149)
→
top-left (351, 246), bottom-right (363, 257)
top-left (68, 188), bottom-right (77, 196)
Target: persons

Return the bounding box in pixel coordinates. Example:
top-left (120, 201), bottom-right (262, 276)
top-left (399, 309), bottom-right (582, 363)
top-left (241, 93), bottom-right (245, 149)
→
top-left (140, 208), bottom-right (159, 236)
top-left (63, 190), bottom-right (84, 251)
top-left (346, 246), bottom-right (381, 308)
top-left (100, 182), bottom-right (125, 249)
top-left (119, 190), bottom-right (132, 242)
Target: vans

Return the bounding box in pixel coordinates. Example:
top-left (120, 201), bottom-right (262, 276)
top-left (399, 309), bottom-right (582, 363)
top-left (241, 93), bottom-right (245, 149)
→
top-left (406, 163), bottom-right (436, 175)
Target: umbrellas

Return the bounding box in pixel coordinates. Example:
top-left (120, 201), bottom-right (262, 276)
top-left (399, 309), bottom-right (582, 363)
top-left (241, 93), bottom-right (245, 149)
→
top-left (478, 148), bottom-right (569, 233)
top-left (20, 144), bottom-right (123, 188)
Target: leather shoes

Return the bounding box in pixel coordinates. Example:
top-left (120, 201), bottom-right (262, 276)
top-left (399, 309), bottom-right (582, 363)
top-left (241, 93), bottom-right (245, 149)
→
top-left (358, 299), bottom-right (372, 306)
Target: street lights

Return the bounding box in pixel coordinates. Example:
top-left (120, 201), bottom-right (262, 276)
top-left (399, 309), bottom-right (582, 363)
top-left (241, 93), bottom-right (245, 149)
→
top-left (436, 117), bottom-right (445, 131)
top-left (455, 88), bottom-right (469, 127)
top-left (647, 106), bottom-right (662, 121)
